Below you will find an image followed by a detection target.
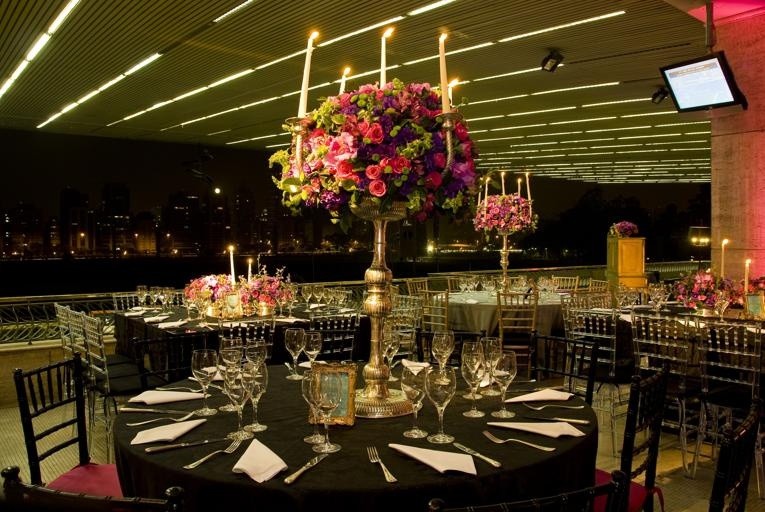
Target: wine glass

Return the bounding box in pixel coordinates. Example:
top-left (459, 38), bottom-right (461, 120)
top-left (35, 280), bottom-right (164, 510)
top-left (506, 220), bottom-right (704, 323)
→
top-left (490, 349), bottom-right (516, 419)
top-left (137, 283), bottom-right (352, 321)
top-left (425, 367), bottom-right (455, 443)
top-left (285, 327), bottom-right (323, 381)
top-left (614, 282), bottom-right (731, 325)
top-left (460, 342), bottom-right (486, 418)
top-left (433, 329), bottom-right (452, 387)
top-left (480, 336), bottom-right (502, 397)
top-left (382, 329), bottom-right (399, 382)
top-left (459, 273), bottom-right (560, 303)
top-left (298, 367), bottom-right (346, 453)
top-left (402, 364), bottom-right (429, 439)
top-left (190, 335), bottom-right (271, 449)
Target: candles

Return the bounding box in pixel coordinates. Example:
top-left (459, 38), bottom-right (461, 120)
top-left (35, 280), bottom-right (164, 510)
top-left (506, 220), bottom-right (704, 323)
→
top-left (477, 170), bottom-right (533, 207)
top-left (297, 29), bottom-right (319, 117)
top-left (338, 67), bottom-right (351, 96)
top-left (438, 32), bottom-right (451, 113)
top-left (448, 78), bottom-right (460, 105)
top-left (379, 26), bottom-right (396, 88)
top-left (721, 238), bottom-right (725, 279)
top-left (744, 263), bottom-right (750, 293)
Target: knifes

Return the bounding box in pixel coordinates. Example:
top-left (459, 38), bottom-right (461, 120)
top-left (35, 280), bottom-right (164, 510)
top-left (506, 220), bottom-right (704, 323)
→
top-left (453, 443), bottom-right (502, 468)
top-left (283, 455), bottom-right (328, 485)
top-left (120, 408), bottom-right (190, 415)
top-left (144, 438), bottom-right (231, 453)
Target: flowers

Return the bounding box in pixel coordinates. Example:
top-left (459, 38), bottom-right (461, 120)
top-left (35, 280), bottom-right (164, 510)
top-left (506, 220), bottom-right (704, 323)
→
top-left (471, 192), bottom-right (539, 236)
top-left (609, 221), bottom-right (639, 237)
top-left (267, 77), bottom-right (480, 238)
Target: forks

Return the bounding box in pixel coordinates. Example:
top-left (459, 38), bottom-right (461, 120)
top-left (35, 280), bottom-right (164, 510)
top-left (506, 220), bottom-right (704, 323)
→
top-left (522, 402), bottom-right (585, 412)
top-left (366, 447), bottom-right (399, 483)
top-left (181, 439), bottom-right (243, 470)
top-left (156, 387), bottom-right (209, 392)
top-left (125, 411), bottom-right (194, 427)
top-left (482, 430), bottom-right (556, 452)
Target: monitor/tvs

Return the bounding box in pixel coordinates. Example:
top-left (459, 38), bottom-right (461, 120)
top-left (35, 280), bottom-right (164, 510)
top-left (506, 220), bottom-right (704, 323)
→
top-left (658, 50), bottom-right (741, 114)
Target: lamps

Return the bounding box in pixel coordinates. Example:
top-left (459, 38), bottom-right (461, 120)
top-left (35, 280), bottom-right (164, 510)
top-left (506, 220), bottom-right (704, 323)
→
top-left (688, 226), bottom-right (711, 249)
